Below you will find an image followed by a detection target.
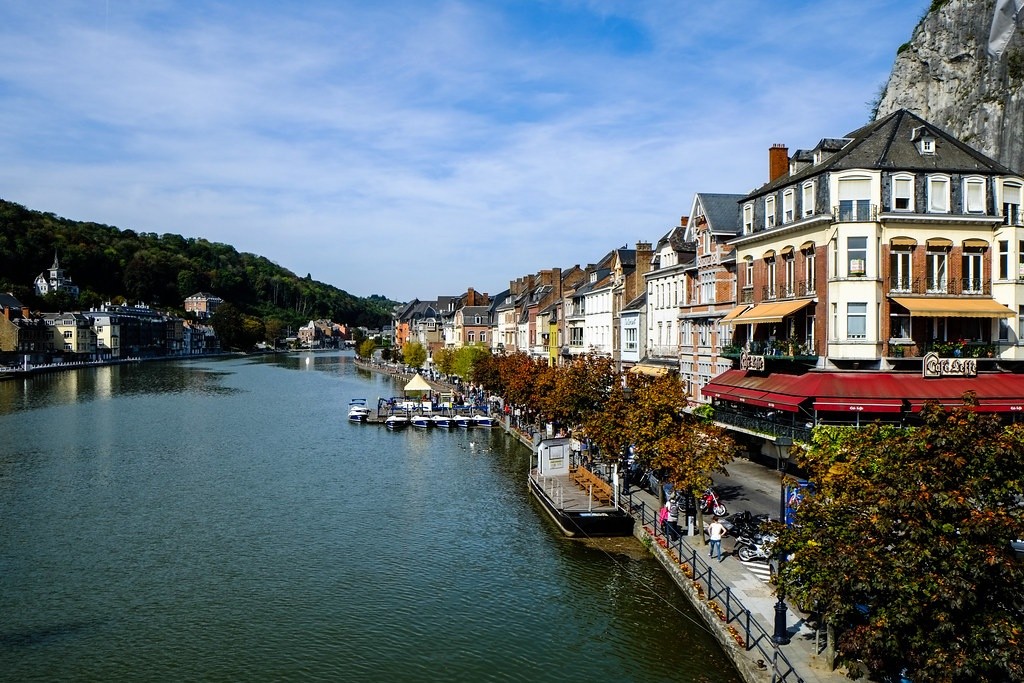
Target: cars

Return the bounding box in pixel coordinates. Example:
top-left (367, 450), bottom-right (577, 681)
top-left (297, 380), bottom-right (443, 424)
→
top-left (648, 468), bottom-right (677, 502)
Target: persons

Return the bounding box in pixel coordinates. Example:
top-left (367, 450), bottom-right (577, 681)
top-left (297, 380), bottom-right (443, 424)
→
top-left (707, 515), bottom-right (727, 562)
top-left (699, 477), bottom-right (718, 513)
top-left (450, 380), bottom-right (588, 465)
top-left (421, 393), bottom-right (439, 407)
top-left (660, 493), bottom-right (679, 541)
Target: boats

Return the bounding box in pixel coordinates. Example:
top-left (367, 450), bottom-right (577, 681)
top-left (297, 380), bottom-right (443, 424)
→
top-left (431, 415), bottom-right (453, 428)
top-left (348, 398), bottom-right (372, 423)
top-left (453, 415), bottom-right (473, 428)
top-left (384, 416), bottom-right (408, 429)
top-left (472, 414), bottom-right (496, 427)
top-left (411, 414), bottom-right (433, 428)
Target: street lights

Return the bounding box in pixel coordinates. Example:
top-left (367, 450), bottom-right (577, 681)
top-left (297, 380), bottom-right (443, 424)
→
top-left (771, 436), bottom-right (797, 646)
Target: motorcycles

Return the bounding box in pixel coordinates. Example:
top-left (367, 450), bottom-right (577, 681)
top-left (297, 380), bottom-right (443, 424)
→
top-left (675, 488), bottom-right (727, 517)
top-left (720, 509), bottom-right (778, 562)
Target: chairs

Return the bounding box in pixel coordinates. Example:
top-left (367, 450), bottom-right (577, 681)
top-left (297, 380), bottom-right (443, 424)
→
top-left (569, 466), bottom-right (613, 508)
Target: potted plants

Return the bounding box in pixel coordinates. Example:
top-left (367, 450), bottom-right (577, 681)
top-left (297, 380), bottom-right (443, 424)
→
top-left (933, 344), bottom-right (953, 358)
top-left (985, 344), bottom-right (996, 358)
top-left (722, 334), bottom-right (807, 356)
top-left (894, 344), bottom-right (905, 358)
top-left (953, 342), bottom-right (964, 358)
top-left (967, 347), bottom-right (985, 358)
top-left (888, 344), bottom-right (894, 357)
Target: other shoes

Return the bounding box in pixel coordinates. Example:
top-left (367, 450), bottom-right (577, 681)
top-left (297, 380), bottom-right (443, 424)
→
top-left (717, 560), bottom-right (721, 563)
top-left (708, 554), bottom-right (712, 557)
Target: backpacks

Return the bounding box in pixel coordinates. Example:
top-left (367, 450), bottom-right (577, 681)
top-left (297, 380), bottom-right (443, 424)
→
top-left (669, 500), bottom-right (679, 517)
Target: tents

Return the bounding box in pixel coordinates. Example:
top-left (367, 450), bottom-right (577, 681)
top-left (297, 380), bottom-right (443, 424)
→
top-left (403, 373), bottom-right (433, 401)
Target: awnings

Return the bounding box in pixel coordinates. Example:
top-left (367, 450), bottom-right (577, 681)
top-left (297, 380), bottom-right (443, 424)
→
top-left (698, 365), bottom-right (1024, 414)
top-left (889, 297), bottom-right (1016, 318)
top-left (718, 299), bottom-right (814, 326)
